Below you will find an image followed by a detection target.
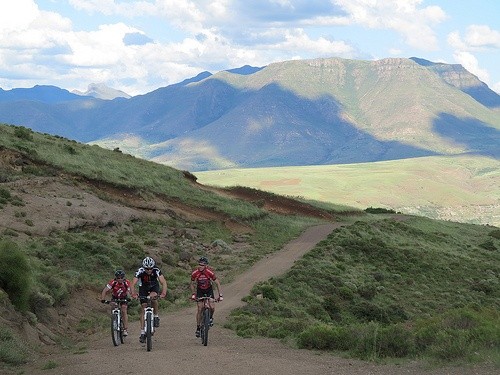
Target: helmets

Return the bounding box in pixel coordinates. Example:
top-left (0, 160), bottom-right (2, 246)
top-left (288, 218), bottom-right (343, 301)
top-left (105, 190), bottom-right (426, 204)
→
top-left (198, 258), bottom-right (208, 264)
top-left (142, 257), bottom-right (155, 268)
top-left (115, 270), bottom-right (125, 276)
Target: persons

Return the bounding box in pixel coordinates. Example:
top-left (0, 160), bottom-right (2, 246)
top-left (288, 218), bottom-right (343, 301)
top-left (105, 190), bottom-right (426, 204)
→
top-left (190, 257), bottom-right (223, 337)
top-left (100, 269), bottom-right (132, 336)
top-left (131, 256), bottom-right (167, 342)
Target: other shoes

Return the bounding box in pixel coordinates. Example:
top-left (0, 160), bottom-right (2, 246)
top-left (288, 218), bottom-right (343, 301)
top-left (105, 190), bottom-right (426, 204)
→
top-left (210, 318), bottom-right (213, 327)
top-left (195, 330), bottom-right (200, 337)
top-left (139, 334), bottom-right (146, 343)
top-left (154, 317), bottom-right (160, 327)
top-left (123, 329), bottom-right (128, 336)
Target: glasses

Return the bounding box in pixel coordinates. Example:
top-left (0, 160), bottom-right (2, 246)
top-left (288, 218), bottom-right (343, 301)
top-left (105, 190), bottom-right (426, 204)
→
top-left (198, 264), bottom-right (205, 266)
top-left (145, 268), bottom-right (153, 270)
top-left (117, 277), bottom-right (123, 279)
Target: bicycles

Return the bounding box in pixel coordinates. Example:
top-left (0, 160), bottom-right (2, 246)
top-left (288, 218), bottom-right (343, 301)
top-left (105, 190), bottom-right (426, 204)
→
top-left (189, 294), bottom-right (223, 346)
top-left (99, 299), bottom-right (131, 346)
top-left (131, 292), bottom-right (165, 351)
